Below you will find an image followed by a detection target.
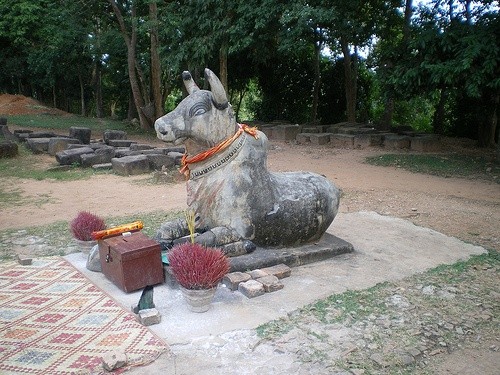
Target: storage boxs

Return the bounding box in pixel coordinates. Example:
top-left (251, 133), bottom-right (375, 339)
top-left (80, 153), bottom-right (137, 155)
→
top-left (98, 231), bottom-right (164, 294)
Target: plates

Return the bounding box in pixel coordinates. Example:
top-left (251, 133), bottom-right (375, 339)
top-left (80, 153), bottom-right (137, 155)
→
top-left (161, 253), bottom-right (170, 265)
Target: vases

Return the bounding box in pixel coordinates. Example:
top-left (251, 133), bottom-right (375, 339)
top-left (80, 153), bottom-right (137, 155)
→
top-left (75, 239), bottom-right (99, 255)
top-left (178, 284), bottom-right (218, 312)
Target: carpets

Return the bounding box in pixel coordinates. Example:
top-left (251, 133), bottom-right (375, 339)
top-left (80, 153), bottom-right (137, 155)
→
top-left (0, 256), bottom-right (178, 375)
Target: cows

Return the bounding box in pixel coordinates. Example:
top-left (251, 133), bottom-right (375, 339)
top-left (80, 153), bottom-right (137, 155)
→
top-left (154, 67), bottom-right (341, 260)
